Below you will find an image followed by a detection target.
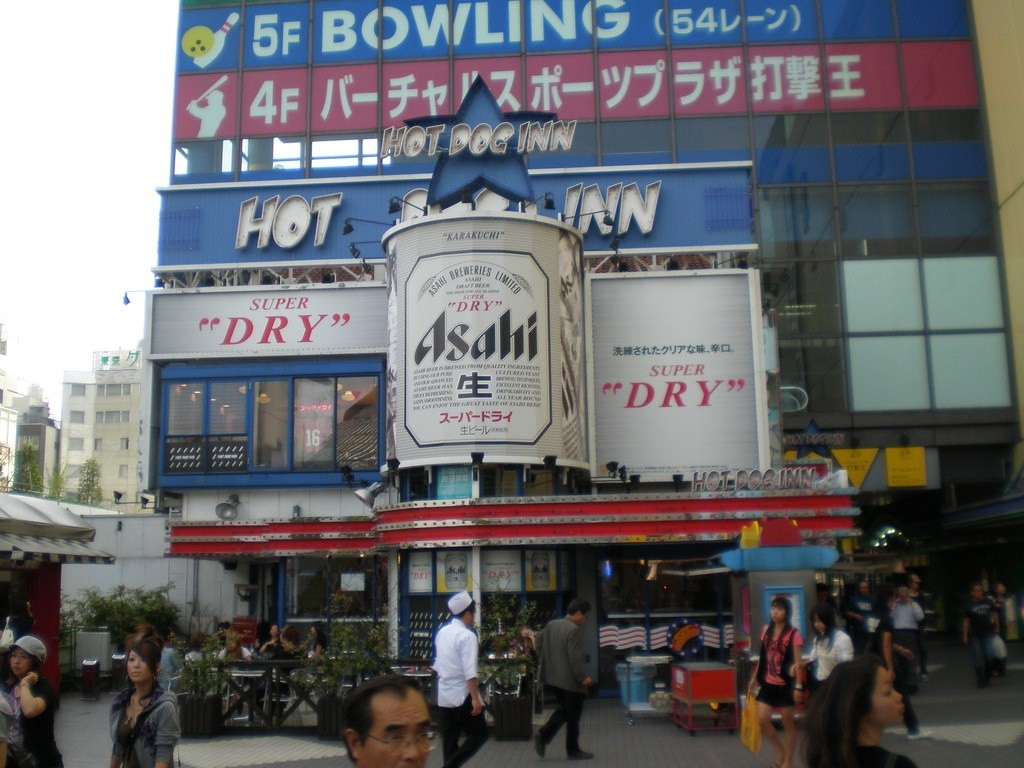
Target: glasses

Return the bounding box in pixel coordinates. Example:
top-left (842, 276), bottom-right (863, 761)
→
top-left (361, 732), bottom-right (439, 753)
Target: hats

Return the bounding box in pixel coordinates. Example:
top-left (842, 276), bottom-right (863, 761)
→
top-left (9, 636), bottom-right (47, 663)
top-left (448, 590), bottom-right (473, 614)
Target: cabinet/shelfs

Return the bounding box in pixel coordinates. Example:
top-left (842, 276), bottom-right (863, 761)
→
top-left (670, 663), bottom-right (739, 735)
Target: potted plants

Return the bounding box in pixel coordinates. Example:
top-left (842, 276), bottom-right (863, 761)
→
top-left (468, 568), bottom-right (542, 741)
top-left (166, 627), bottom-right (249, 740)
top-left (285, 592), bottom-right (396, 740)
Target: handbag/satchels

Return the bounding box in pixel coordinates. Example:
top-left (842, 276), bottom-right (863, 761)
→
top-left (0, 617), bottom-right (15, 652)
top-left (12, 749), bottom-right (40, 768)
top-left (740, 692), bottom-right (762, 753)
top-left (989, 635), bottom-right (1009, 659)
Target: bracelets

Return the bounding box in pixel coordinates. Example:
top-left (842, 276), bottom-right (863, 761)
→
top-left (20, 683), bottom-right (31, 691)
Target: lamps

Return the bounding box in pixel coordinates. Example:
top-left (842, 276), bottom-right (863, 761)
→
top-left (714, 254), bottom-right (748, 270)
top-left (461, 186), bottom-right (473, 203)
top-left (582, 233), bottom-right (619, 250)
top-left (354, 479), bottom-right (389, 507)
top-left (561, 210), bottom-right (614, 226)
top-left (901, 433), bottom-right (911, 444)
top-left (123, 291), bottom-right (145, 305)
top-left (222, 562), bottom-right (237, 570)
top-left (525, 455), bottom-right (558, 482)
top-left (521, 192), bottom-right (556, 212)
top-left (586, 461), bottom-right (618, 479)
top-left (140, 497), bottom-right (156, 509)
top-left (322, 270), bottom-right (335, 283)
top-left (673, 474), bottom-right (683, 492)
top-left (630, 476), bottom-right (640, 490)
top-left (611, 249), bottom-right (629, 273)
top-left (617, 466), bottom-right (630, 493)
top-left (361, 257), bottom-right (385, 274)
top-left (388, 195), bottom-right (428, 217)
top-left (215, 494), bottom-right (240, 520)
top-left (387, 459), bottom-right (425, 473)
top-left (349, 240), bottom-right (381, 258)
top-left (471, 452), bottom-right (485, 481)
top-left (115, 491), bottom-right (157, 505)
top-left (851, 438), bottom-right (861, 448)
top-left (262, 269), bottom-right (280, 285)
top-left (204, 271), bottom-right (223, 286)
top-left (343, 217), bottom-right (397, 235)
top-left (340, 465), bottom-right (354, 485)
top-left (667, 256), bottom-right (679, 270)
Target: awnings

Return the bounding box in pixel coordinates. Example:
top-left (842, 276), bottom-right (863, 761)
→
top-left (830, 562), bottom-right (904, 572)
top-left (0, 531), bottom-right (116, 564)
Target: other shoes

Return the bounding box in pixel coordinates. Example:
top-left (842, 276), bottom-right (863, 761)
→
top-left (908, 730), bottom-right (932, 740)
top-left (535, 734), bottom-right (546, 758)
top-left (567, 751), bottom-right (593, 760)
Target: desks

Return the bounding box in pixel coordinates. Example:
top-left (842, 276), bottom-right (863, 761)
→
top-left (198, 668), bottom-right (346, 728)
top-left (391, 666), bottom-right (433, 679)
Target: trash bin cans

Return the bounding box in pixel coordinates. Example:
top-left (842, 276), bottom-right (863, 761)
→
top-left (616, 663), bottom-right (657, 707)
top-left (80, 660), bottom-right (101, 700)
top-left (496, 673), bottom-right (532, 742)
top-left (111, 654), bottom-right (127, 692)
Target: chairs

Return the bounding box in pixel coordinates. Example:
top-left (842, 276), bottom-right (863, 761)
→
top-left (486, 653), bottom-right (522, 703)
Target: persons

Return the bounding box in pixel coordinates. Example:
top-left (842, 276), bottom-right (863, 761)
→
top-left (157, 627), bottom-right (209, 692)
top-left (818, 574), bottom-right (929, 739)
top-left (1, 636), bottom-right (64, 768)
top-left (800, 661), bottom-right (917, 768)
top-left (535, 599), bottom-right (594, 759)
top-left (341, 674), bottom-right (431, 768)
top-left (789, 604), bottom-right (854, 695)
top-left (215, 622), bottom-right (328, 720)
top-left (433, 590), bottom-right (489, 768)
top-left (963, 583), bottom-right (1007, 687)
top-left (110, 625), bottom-right (181, 768)
top-left (748, 597), bottom-right (804, 768)
top-left (0, 593), bottom-right (36, 684)
